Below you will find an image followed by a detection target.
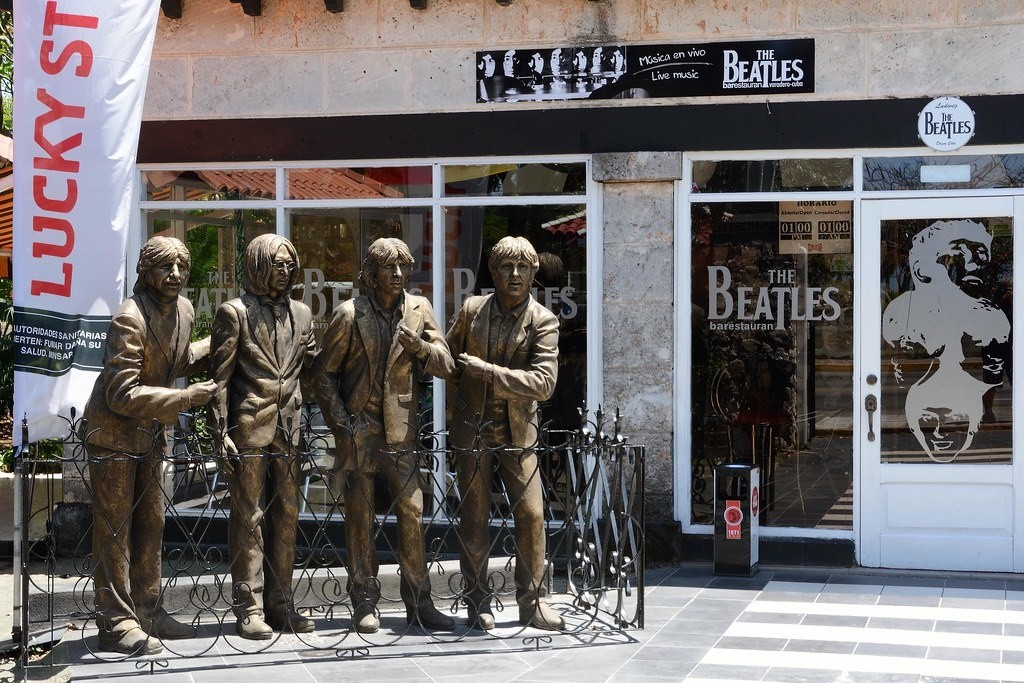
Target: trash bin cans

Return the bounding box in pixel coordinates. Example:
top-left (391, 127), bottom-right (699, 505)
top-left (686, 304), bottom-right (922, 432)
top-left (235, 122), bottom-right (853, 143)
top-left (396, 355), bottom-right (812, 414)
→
top-left (714, 463), bottom-right (760, 580)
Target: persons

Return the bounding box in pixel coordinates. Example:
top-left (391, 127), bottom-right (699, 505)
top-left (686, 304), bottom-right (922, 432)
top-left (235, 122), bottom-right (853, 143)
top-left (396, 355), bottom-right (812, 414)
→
top-left (78, 233), bottom-right (566, 654)
